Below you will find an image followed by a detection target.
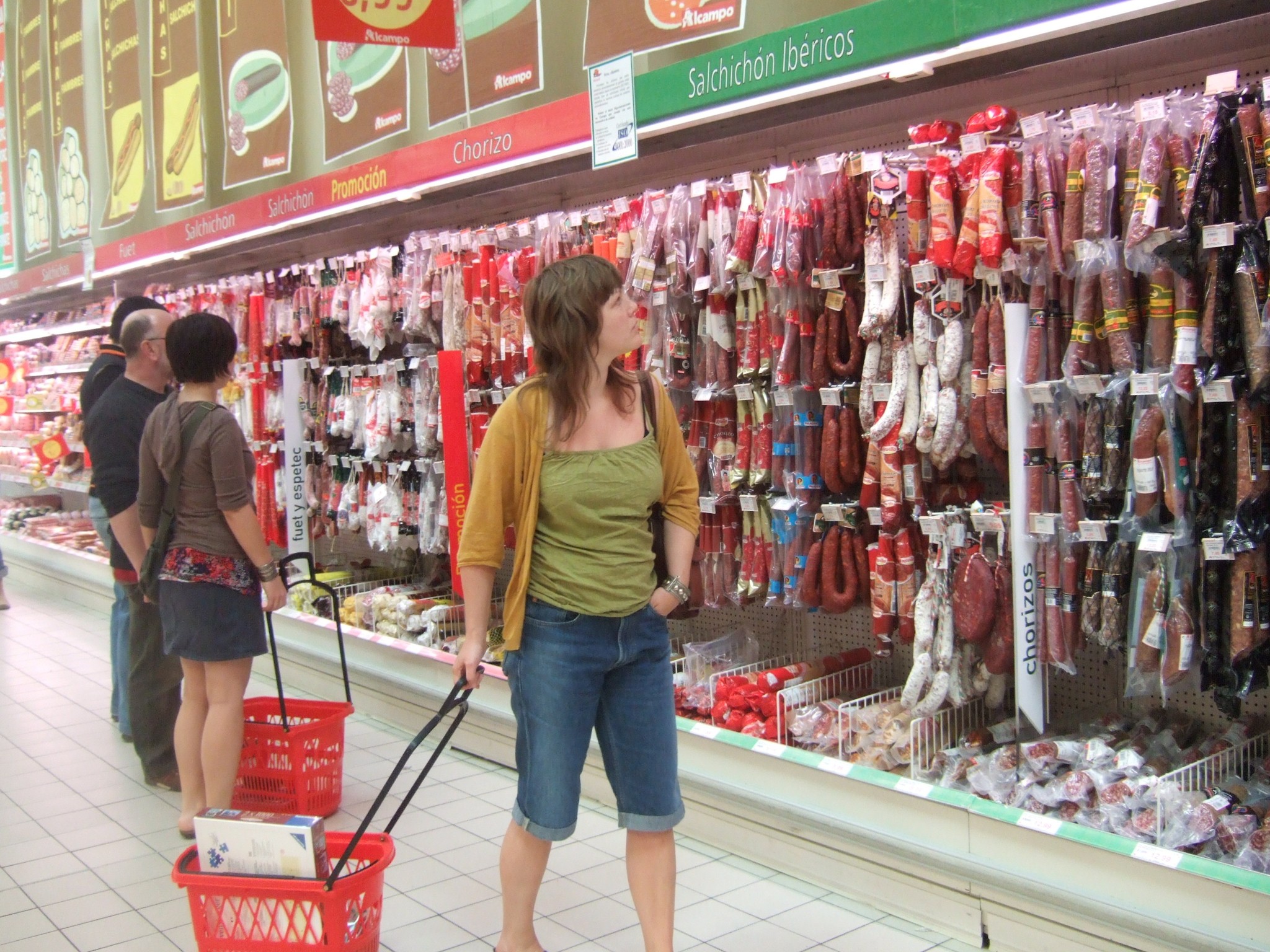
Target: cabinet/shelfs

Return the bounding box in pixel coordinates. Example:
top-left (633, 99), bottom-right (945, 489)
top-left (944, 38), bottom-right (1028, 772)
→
top-left (0, 319), bottom-right (111, 566)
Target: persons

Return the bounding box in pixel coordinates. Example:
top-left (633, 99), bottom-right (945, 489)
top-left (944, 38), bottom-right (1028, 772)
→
top-left (138, 312), bottom-right (287, 838)
top-left (454, 255), bottom-right (704, 952)
top-left (80, 295), bottom-right (179, 792)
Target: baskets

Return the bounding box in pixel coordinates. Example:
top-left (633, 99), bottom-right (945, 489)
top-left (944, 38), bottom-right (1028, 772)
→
top-left (171, 831), bottom-right (395, 952)
top-left (231, 696), bottom-right (354, 819)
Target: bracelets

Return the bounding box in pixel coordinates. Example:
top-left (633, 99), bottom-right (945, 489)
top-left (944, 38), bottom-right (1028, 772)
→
top-left (255, 559), bottom-right (278, 583)
top-left (659, 574), bottom-right (692, 605)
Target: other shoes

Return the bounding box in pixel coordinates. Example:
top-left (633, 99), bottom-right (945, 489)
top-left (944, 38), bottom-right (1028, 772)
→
top-left (112, 715), bottom-right (119, 722)
top-left (122, 733), bottom-right (134, 742)
top-left (146, 770), bottom-right (181, 792)
top-left (180, 829), bottom-right (195, 839)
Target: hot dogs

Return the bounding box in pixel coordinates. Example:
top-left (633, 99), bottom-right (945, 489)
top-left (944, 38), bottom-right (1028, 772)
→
top-left (114, 112), bottom-right (141, 196)
top-left (165, 84), bottom-right (200, 175)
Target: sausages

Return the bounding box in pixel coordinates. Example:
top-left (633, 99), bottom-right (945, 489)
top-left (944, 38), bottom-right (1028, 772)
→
top-left (329, 71), bottom-right (354, 116)
top-left (214, 201), bottom-right (632, 654)
top-left (230, 111), bottom-right (245, 150)
top-left (429, 26), bottom-right (462, 74)
top-left (235, 64), bottom-right (281, 101)
top-left (337, 42), bottom-right (365, 60)
top-left (624, 81), bottom-right (1270, 867)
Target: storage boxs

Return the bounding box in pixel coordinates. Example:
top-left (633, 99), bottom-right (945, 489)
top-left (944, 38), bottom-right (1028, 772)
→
top-left (193, 806), bottom-right (329, 944)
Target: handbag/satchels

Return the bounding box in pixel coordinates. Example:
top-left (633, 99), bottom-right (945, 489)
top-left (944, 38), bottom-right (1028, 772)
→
top-left (637, 369), bottom-right (704, 619)
top-left (137, 542), bottom-right (172, 602)
